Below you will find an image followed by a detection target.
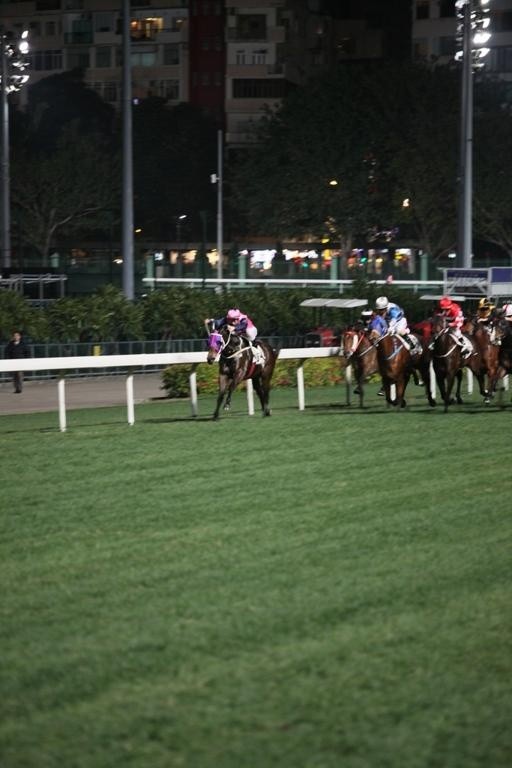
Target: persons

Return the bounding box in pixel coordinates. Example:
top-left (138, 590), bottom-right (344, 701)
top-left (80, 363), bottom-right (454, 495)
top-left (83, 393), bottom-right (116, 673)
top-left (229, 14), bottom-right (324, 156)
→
top-left (3, 331), bottom-right (32, 394)
top-left (204, 306), bottom-right (259, 361)
top-left (358, 294), bottom-right (512, 357)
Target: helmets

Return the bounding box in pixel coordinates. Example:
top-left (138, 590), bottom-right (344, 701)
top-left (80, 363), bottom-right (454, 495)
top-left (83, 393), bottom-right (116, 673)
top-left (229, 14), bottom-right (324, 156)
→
top-left (226, 308), bottom-right (240, 320)
top-left (439, 298), bottom-right (452, 310)
top-left (375, 296), bottom-right (389, 310)
top-left (479, 298), bottom-right (488, 308)
top-left (359, 308), bottom-right (374, 321)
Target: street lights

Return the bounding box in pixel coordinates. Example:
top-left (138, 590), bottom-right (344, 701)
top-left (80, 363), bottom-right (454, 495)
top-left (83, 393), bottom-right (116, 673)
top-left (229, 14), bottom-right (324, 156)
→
top-left (0, 27), bottom-right (32, 266)
top-left (175, 213), bottom-right (188, 280)
top-left (453, 0), bottom-right (495, 270)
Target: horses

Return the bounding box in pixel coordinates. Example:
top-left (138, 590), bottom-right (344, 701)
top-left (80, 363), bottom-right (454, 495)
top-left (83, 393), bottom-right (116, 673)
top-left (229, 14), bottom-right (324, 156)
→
top-left (343, 309), bottom-right (512, 413)
top-left (207, 326), bottom-right (283, 421)
